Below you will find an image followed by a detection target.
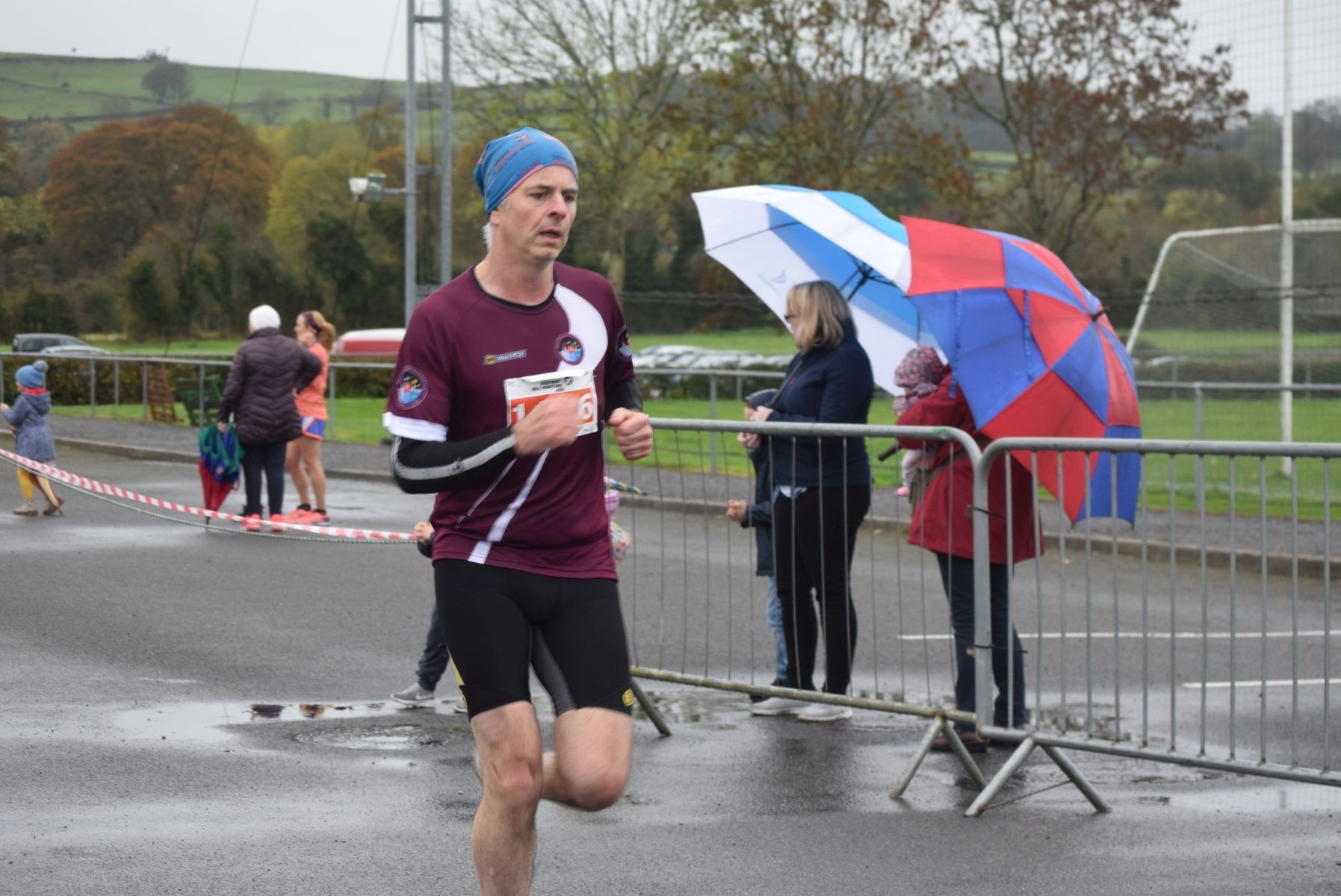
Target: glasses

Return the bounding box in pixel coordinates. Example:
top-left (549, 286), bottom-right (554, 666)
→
top-left (784, 314), bottom-right (795, 321)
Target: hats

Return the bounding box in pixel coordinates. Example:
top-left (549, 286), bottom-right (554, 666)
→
top-left (15, 360), bottom-right (48, 388)
top-left (473, 127), bottom-right (579, 221)
top-left (248, 305), bottom-right (281, 330)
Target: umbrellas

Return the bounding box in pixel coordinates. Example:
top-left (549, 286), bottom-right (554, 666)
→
top-left (879, 216), bottom-right (1143, 530)
top-left (9, 424), bottom-right (63, 515)
top-left (198, 420), bottom-right (244, 531)
top-left (690, 182), bottom-right (946, 408)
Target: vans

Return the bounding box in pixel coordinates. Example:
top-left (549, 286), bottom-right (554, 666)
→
top-left (332, 329), bottom-right (408, 354)
top-left (13, 332), bottom-right (92, 353)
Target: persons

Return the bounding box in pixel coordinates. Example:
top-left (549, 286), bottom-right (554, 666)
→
top-left (383, 125), bottom-right (654, 896)
top-left (896, 364), bottom-right (1044, 752)
top-left (391, 521), bottom-right (467, 713)
top-left (216, 303), bottom-right (322, 531)
top-left (726, 389), bottom-right (789, 702)
top-left (0, 360), bottom-right (63, 515)
top-left (893, 346), bottom-right (943, 496)
top-left (736, 280), bottom-right (875, 721)
top-left (284, 310), bottom-right (337, 526)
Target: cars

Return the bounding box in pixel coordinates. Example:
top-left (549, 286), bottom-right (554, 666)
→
top-left (41, 344), bottom-right (111, 357)
top-left (632, 343), bottom-right (796, 372)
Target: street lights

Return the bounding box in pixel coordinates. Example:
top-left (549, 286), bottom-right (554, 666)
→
top-left (347, 169), bottom-right (417, 329)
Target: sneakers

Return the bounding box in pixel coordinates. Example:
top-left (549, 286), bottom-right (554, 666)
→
top-left (799, 703), bottom-right (853, 721)
top-left (239, 514), bottom-right (261, 531)
top-left (283, 508), bottom-right (312, 523)
top-left (270, 514), bottom-right (284, 532)
top-left (392, 681), bottom-right (435, 709)
top-left (295, 512), bottom-right (330, 526)
top-left (14, 501), bottom-right (38, 515)
top-left (749, 697), bottom-right (812, 714)
top-left (453, 702), bottom-right (467, 713)
top-left (44, 496), bottom-right (65, 515)
top-left (934, 731), bottom-right (985, 749)
